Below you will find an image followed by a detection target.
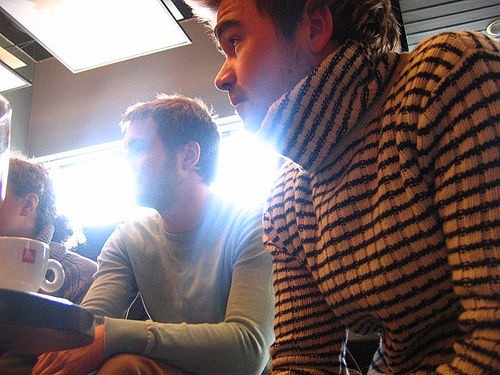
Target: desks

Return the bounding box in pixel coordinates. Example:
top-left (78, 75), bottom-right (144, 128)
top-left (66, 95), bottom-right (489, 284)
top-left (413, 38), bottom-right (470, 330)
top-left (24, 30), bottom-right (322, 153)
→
top-left (0, 288), bottom-right (96, 375)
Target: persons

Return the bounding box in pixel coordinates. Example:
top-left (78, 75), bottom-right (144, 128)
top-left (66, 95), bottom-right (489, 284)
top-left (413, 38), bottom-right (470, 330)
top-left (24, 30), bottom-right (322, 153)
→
top-left (0, 155), bottom-right (99, 375)
top-left (195, 0), bottom-right (500, 375)
top-left (31, 91), bottom-right (276, 375)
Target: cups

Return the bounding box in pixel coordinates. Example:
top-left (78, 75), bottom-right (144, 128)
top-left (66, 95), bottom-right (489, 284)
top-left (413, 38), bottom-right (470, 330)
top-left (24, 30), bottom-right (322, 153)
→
top-left (0, 236), bottom-right (65, 293)
